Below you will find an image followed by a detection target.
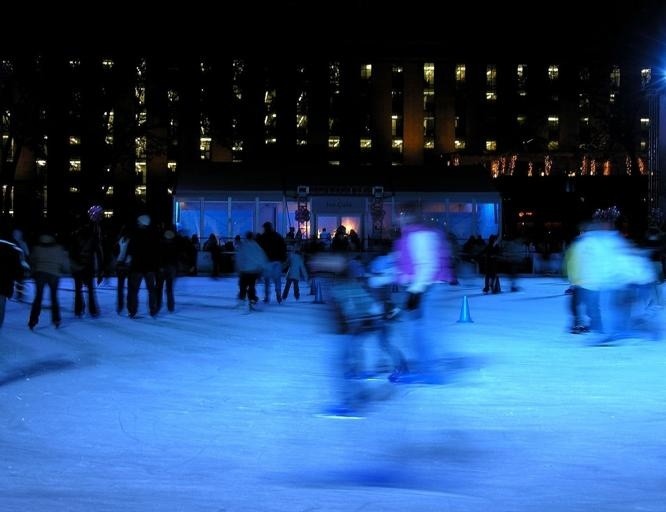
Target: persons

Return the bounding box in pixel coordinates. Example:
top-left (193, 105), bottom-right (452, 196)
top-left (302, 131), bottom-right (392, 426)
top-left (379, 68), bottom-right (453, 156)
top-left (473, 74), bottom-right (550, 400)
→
top-left (301, 250), bottom-right (383, 425)
top-left (203, 218), bottom-right (462, 322)
top-left (462, 220), bottom-right (666, 343)
top-left (1, 202), bottom-right (202, 331)
top-left (344, 254), bottom-right (410, 383)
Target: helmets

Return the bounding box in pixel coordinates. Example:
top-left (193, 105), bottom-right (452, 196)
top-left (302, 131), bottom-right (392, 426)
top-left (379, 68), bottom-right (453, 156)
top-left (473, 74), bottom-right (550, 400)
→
top-left (88, 205), bottom-right (103, 221)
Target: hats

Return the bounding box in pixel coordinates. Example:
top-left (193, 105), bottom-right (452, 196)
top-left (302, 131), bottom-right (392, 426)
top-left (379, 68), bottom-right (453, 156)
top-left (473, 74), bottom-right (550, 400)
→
top-left (164, 230), bottom-right (175, 239)
top-left (137, 215), bottom-right (150, 226)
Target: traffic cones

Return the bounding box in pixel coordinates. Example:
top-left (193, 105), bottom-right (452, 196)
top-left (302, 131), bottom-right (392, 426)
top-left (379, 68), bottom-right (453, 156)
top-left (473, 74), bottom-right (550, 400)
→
top-left (456, 296), bottom-right (474, 324)
top-left (312, 282), bottom-right (326, 304)
top-left (307, 277), bottom-right (319, 296)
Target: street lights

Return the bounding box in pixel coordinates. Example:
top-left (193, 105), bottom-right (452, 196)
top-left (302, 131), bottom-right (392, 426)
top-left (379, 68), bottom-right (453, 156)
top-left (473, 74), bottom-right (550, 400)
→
top-left (372, 186), bottom-right (386, 241)
top-left (292, 183), bottom-right (311, 239)
